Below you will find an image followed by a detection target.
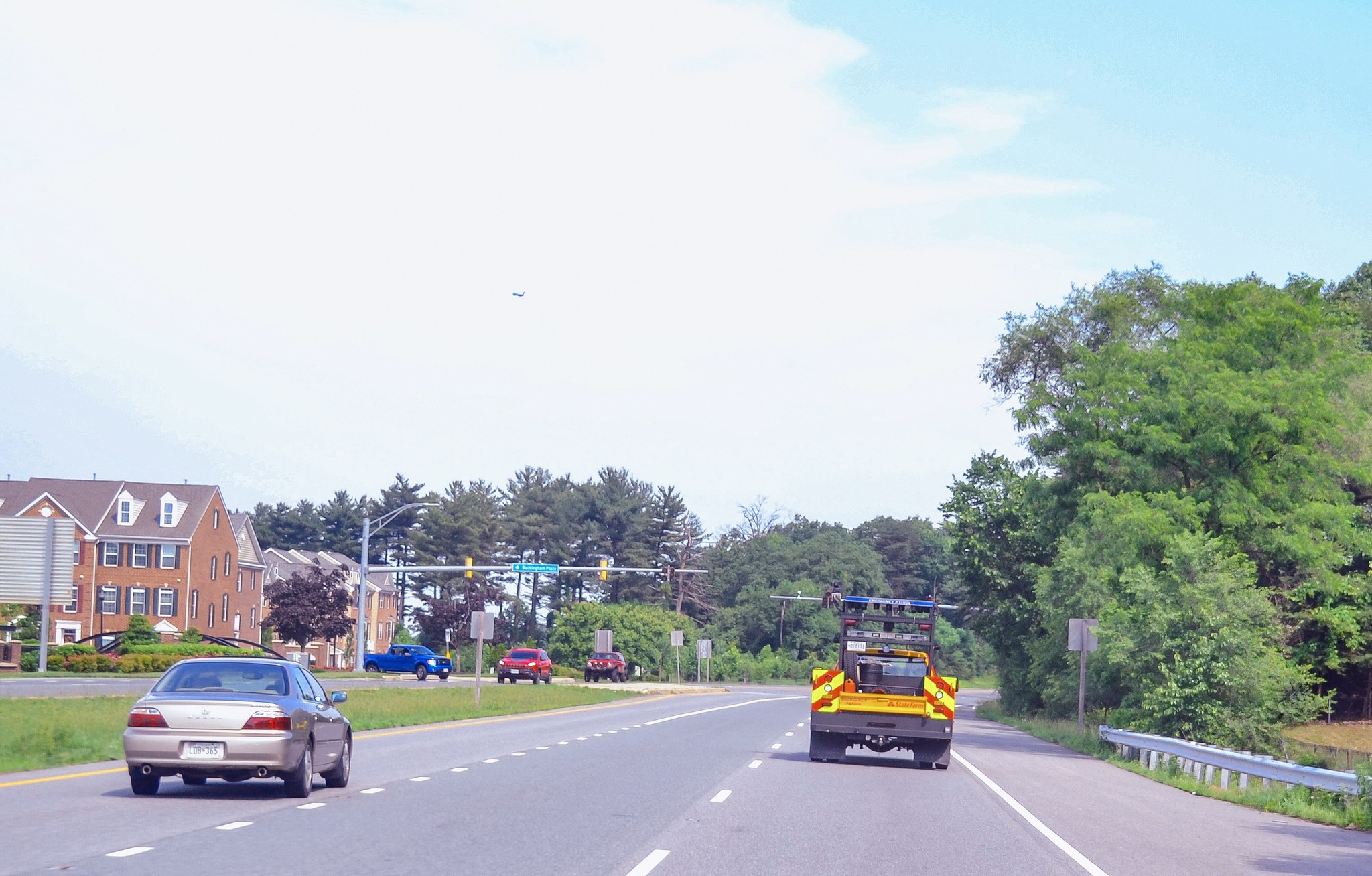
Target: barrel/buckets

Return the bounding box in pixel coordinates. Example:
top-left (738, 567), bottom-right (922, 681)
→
top-left (860, 664), bottom-right (883, 684)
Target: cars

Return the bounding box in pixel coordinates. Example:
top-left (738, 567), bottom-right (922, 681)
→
top-left (123, 658), bottom-right (354, 797)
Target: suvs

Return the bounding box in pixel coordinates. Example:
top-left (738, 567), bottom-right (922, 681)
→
top-left (498, 647), bottom-right (554, 685)
top-left (584, 651), bottom-right (628, 684)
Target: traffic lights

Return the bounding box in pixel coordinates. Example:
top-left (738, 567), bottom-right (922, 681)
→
top-left (926, 596), bottom-right (934, 614)
top-left (660, 566), bottom-right (675, 583)
top-left (821, 581), bottom-right (841, 609)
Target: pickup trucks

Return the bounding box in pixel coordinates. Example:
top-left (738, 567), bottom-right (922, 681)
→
top-left (363, 644), bottom-right (454, 681)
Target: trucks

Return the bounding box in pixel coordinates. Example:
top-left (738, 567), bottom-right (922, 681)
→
top-left (808, 590), bottom-right (959, 770)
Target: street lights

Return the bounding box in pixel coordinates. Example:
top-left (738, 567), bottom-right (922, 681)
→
top-left (354, 502), bottom-right (440, 671)
top-left (99, 589), bottom-right (106, 634)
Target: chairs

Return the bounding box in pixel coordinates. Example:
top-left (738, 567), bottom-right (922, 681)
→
top-left (265, 679), bottom-right (284, 693)
top-left (183, 674), bottom-right (222, 689)
top-left (402, 648), bottom-right (410, 655)
